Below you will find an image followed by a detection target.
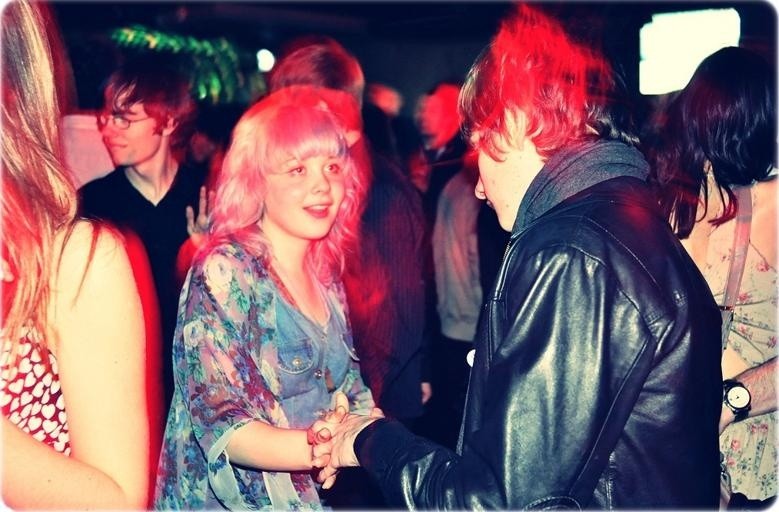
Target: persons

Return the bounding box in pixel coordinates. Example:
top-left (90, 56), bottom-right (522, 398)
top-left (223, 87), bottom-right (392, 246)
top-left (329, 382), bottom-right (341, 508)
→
top-left (269, 41), bottom-right (395, 408)
top-left (1, 0), bottom-right (163, 510)
top-left (645, 90), bottom-right (778, 512)
top-left (309, 12), bottom-right (720, 511)
top-left (76, 56), bottom-right (215, 431)
top-left (363, 83), bottom-right (501, 418)
top-left (155, 85), bottom-right (382, 511)
top-left (682, 46), bottom-right (779, 435)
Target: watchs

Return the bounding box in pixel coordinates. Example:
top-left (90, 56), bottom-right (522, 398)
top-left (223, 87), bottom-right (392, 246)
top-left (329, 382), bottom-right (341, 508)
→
top-left (722, 376), bottom-right (752, 425)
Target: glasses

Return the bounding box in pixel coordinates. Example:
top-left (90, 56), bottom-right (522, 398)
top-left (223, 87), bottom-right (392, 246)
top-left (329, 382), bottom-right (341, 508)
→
top-left (97, 111), bottom-right (148, 131)
